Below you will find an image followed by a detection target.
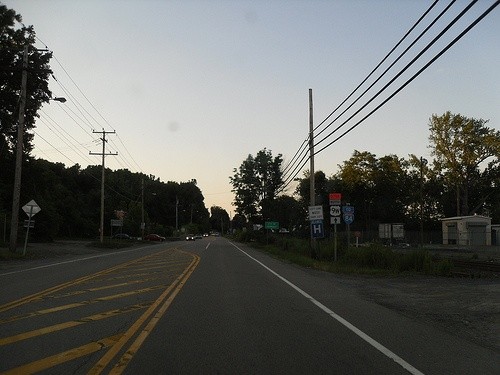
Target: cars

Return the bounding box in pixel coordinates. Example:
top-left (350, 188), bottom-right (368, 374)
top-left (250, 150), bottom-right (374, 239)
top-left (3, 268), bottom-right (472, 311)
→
top-left (209, 231), bottom-right (219, 236)
top-left (203, 233), bottom-right (209, 237)
top-left (112, 233), bottom-right (132, 240)
top-left (185, 233), bottom-right (195, 241)
top-left (143, 234), bottom-right (166, 242)
top-left (398, 243), bottom-right (410, 249)
top-left (194, 234), bottom-right (202, 239)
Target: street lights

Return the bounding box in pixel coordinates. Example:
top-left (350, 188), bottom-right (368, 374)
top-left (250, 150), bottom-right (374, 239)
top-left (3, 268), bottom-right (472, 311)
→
top-left (8, 97), bottom-right (67, 255)
